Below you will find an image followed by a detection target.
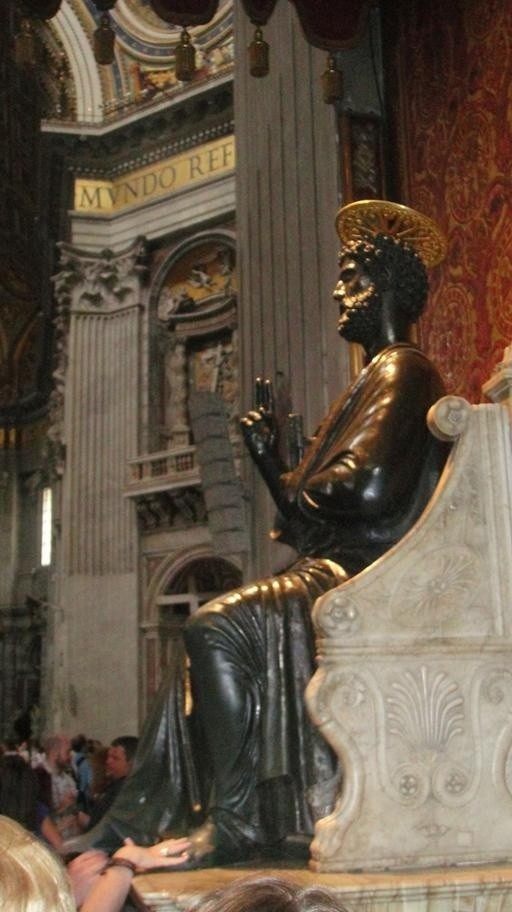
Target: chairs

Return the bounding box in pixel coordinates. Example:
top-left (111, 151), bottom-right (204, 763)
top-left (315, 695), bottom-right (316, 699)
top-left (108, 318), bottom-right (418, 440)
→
top-left (309, 348), bottom-right (512, 876)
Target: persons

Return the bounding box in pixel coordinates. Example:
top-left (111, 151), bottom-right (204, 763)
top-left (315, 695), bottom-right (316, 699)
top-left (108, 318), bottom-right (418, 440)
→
top-left (185, 869), bottom-right (349, 912)
top-left (53, 197), bottom-right (448, 873)
top-left (1, 730), bottom-right (194, 912)
